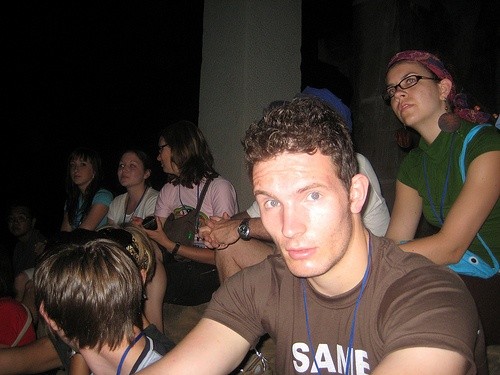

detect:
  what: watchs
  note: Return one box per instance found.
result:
[239,217,251,241]
[64,347,80,358]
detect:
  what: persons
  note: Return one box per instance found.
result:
[141,117,240,334]
[15,145,114,317]
[33,238,177,375]
[36,142,159,340]
[0,223,157,375]
[198,87,391,286]
[1,188,44,300]
[383,49,500,347]
[126,93,488,375]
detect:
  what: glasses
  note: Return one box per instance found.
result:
[382,75,442,102]
[157,144,169,153]
[6,216,32,223]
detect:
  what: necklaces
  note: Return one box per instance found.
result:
[128,192,139,212]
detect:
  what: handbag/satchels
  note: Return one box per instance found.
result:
[400,240,495,279]
[162,204,208,262]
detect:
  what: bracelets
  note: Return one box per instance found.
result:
[171,241,183,255]
